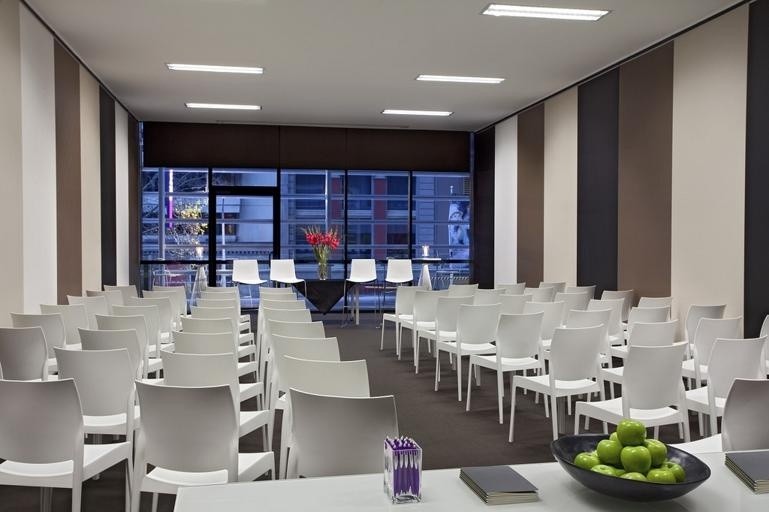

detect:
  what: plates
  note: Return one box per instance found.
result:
[550,433,711,502]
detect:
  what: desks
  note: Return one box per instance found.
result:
[171,449,769,512]
[411,257,442,290]
[292,278,355,328]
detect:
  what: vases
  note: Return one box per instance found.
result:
[318,262,328,281]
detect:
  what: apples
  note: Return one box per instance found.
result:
[574,420,685,484]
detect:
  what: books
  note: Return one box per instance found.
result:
[725,451,769,495]
[459,466,540,506]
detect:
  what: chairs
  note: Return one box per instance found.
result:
[338,258,380,330]
[230,258,267,307]
[381,258,414,314]
[267,258,309,301]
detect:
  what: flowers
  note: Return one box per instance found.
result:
[299,223,343,278]
